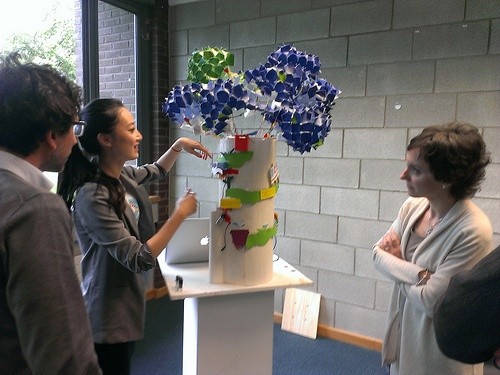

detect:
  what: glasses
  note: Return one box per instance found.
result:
[71,119,87,137]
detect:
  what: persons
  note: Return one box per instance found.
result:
[432,243,499,363]
[0,51,103,375]
[371,121,493,374]
[57,99,213,374]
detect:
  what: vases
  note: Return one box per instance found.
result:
[209,137,278,286]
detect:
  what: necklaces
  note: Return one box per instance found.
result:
[422,211,444,235]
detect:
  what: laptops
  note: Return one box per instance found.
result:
[164,218,209,264]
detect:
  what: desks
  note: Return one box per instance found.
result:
[156,247,313,374]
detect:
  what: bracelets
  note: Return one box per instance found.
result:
[416,270,431,286]
[171,144,183,153]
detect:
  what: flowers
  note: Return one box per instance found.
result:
[163,44,341,156]
